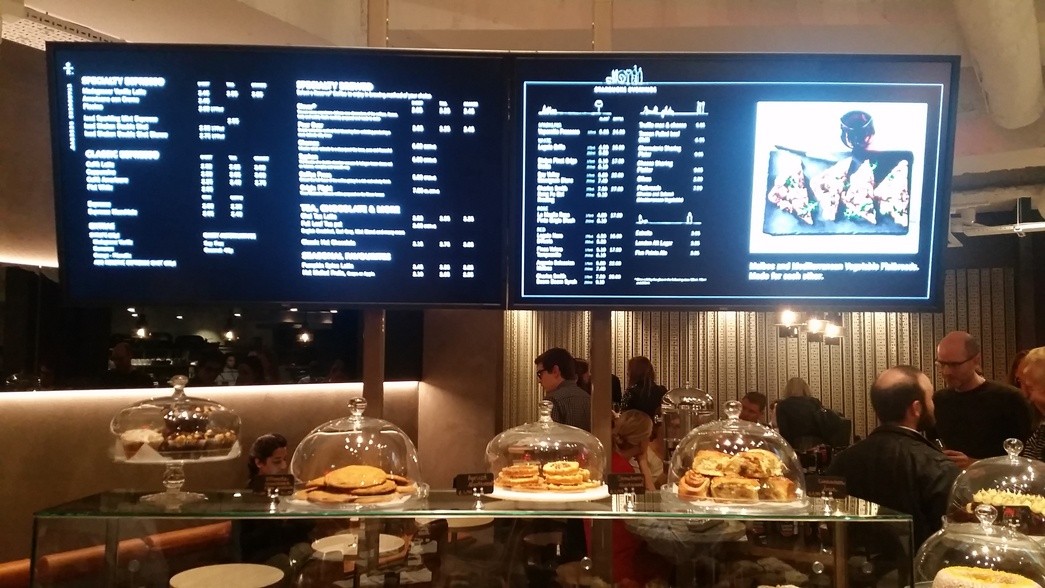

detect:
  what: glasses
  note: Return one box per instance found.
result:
[934,354,976,370]
[536,364,564,381]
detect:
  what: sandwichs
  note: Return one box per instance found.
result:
[767,156,908,226]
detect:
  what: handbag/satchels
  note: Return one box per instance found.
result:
[812,396,843,441]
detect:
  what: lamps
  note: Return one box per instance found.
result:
[773,310,844,347]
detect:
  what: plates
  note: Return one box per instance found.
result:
[413,517,494,528]
[311,534,405,557]
[523,532,564,545]
[763,151,913,235]
[169,563,284,588]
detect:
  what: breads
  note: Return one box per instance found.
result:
[494,461,599,491]
[678,449,792,504]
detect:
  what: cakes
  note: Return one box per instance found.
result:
[966,489,1045,538]
[931,565,1044,588]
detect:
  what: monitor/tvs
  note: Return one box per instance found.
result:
[47,38,509,313]
[510,51,960,315]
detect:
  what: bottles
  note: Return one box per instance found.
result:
[751,521,819,539]
[796,444,849,475]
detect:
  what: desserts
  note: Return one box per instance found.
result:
[119,409,238,460]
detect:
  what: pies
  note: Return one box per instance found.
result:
[296,464,418,503]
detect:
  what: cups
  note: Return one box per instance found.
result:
[841,110,874,152]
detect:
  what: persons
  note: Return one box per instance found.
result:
[228,433,317,565]
[612,355,682,495]
[1006,346,1045,462]
[844,365,958,554]
[740,376,862,498]
[535,348,590,434]
[921,332,1035,472]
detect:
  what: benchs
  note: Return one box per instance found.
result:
[0,522,234,588]
